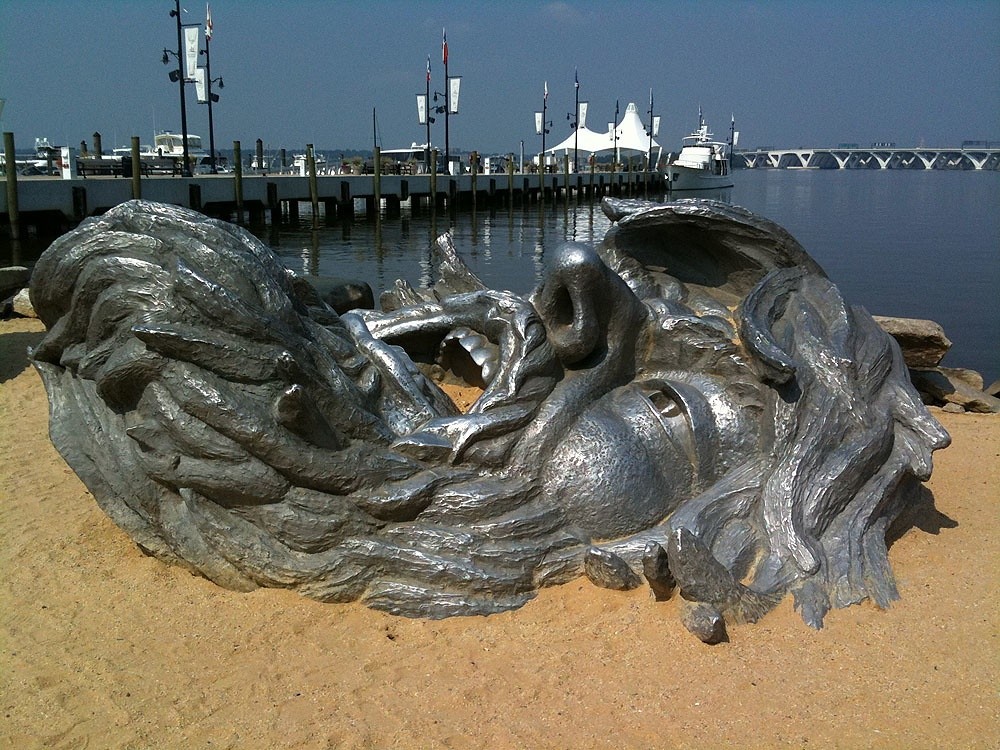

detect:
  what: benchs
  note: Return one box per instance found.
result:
[76,158,124,178]
[140,158,183,178]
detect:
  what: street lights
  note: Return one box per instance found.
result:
[611,100,622,163]
[567,67,580,174]
[540,80,553,155]
[726,114,734,168]
[434,29,458,175]
[196,2,225,175]
[642,88,657,171]
[423,54,435,175]
[160,0,202,178]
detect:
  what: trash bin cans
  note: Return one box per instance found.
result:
[121,156,133,177]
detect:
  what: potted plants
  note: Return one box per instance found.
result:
[340,157,364,175]
[406,160,417,175]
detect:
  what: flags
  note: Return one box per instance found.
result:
[426,54,431,82]
[205,3,213,41]
[575,67,579,88]
[615,97,619,114]
[443,27,449,65]
[650,88,654,104]
[731,113,735,126]
[544,81,548,101]
[698,104,702,118]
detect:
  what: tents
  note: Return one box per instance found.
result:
[539,103,660,174]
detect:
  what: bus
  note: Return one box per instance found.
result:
[871,141,895,148]
[732,148,749,153]
[838,142,858,149]
[757,144,776,151]
[961,141,987,148]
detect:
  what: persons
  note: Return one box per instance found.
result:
[32,196,952,646]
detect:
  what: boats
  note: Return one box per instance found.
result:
[667,120,736,190]
